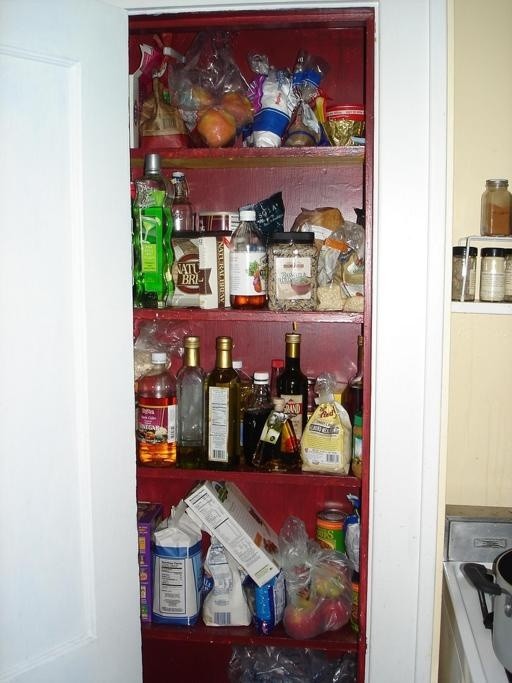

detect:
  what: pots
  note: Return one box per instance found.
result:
[462,548,512,673]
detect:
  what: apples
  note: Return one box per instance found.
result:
[219,93,251,124]
[315,565,347,601]
[286,564,311,601]
[284,600,322,639]
[322,595,352,630]
[190,85,212,115]
[198,106,237,146]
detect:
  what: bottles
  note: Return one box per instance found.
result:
[480,247,507,305]
[179,336,209,466]
[319,508,349,557]
[480,177,511,239]
[134,152,173,309]
[137,355,178,466]
[173,172,193,233]
[208,335,240,471]
[454,244,478,302]
[234,361,254,403]
[227,210,266,308]
[269,227,320,310]
[250,374,270,447]
[268,358,284,394]
[342,332,366,475]
[279,330,308,451]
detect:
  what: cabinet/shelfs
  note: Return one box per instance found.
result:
[451,235,512,314]
[438,590,465,683]
[127,6,374,682]
